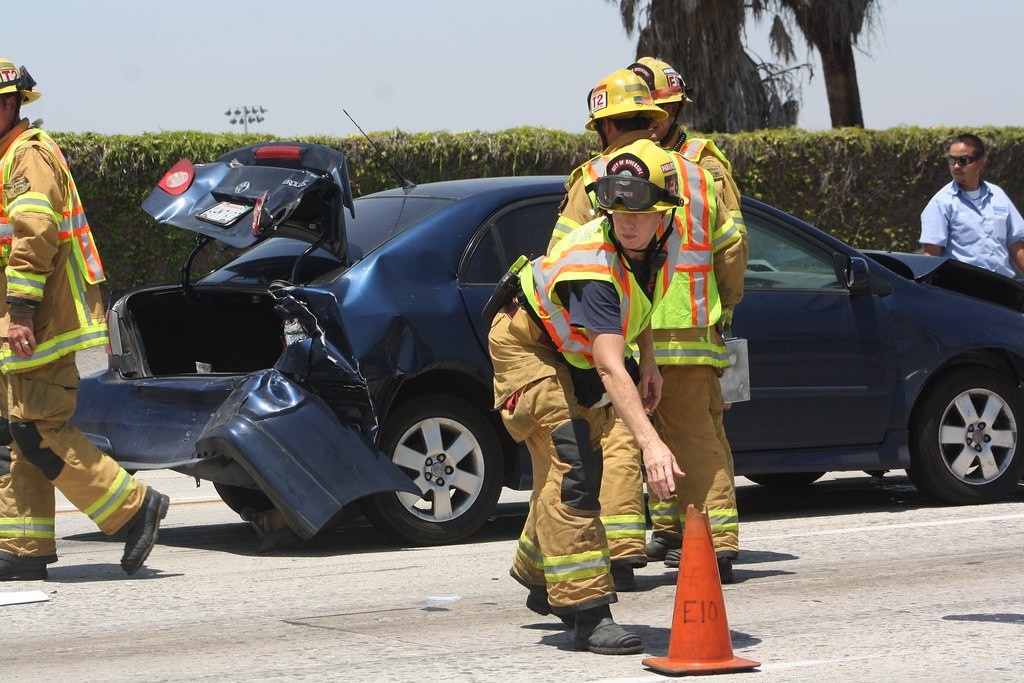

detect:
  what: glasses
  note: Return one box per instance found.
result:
[946,154,980,166]
[594,176,669,211]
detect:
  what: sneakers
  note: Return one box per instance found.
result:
[717,558,734,583]
[0,559,48,580]
[575,605,644,655]
[664,548,682,567]
[645,540,678,562]
[526,590,575,629]
[610,564,636,591]
[121,488,170,575]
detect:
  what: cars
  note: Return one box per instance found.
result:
[71,141,1024,549]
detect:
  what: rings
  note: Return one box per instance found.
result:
[22,341,28,347]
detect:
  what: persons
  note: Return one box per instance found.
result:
[919,134,1024,282]
[547,56,748,582]
[0,57,170,582]
[484,175,686,653]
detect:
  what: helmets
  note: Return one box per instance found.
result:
[585,70,669,131]
[596,139,690,213]
[626,57,693,105]
[0,58,41,106]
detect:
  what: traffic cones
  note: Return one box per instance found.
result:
[638,501,762,676]
